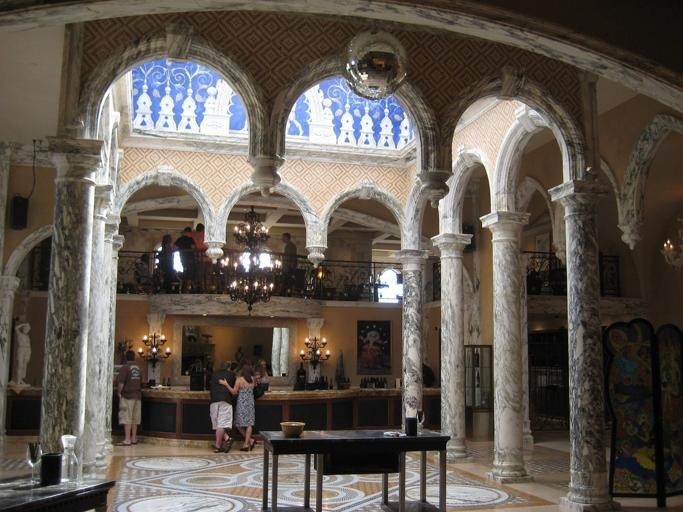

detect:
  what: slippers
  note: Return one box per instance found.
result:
[114,439,141,447]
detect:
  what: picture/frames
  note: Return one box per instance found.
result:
[355,319,393,377]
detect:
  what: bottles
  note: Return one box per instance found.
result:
[314,376,386,390]
[161,377,171,390]
[59,434,78,483]
[296,361,306,391]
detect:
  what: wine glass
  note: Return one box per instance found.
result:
[25,439,41,485]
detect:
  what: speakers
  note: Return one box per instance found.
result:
[463,225,476,253]
[10,196,28,231]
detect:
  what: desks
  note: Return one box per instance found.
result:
[259,429,451,512]
[0,473,116,512]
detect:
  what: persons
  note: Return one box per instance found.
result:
[134,253,152,285]
[218,363,259,452]
[422,361,435,386]
[115,349,143,447]
[13,322,32,386]
[206,362,235,454]
[361,330,388,372]
[235,258,244,286]
[280,232,298,296]
[256,358,268,376]
[172,226,196,294]
[159,234,174,293]
[177,222,209,293]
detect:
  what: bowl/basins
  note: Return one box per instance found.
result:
[279,421,306,438]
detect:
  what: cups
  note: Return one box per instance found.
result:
[405,416,417,436]
[41,451,61,486]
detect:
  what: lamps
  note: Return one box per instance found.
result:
[138,332,172,372]
[660,217,683,272]
[300,336,331,374]
[220,205,281,307]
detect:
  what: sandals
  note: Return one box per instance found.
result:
[213,437,233,452]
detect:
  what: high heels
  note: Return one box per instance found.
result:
[240,440,257,451]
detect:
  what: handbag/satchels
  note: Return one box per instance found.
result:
[254,384,264,398]
